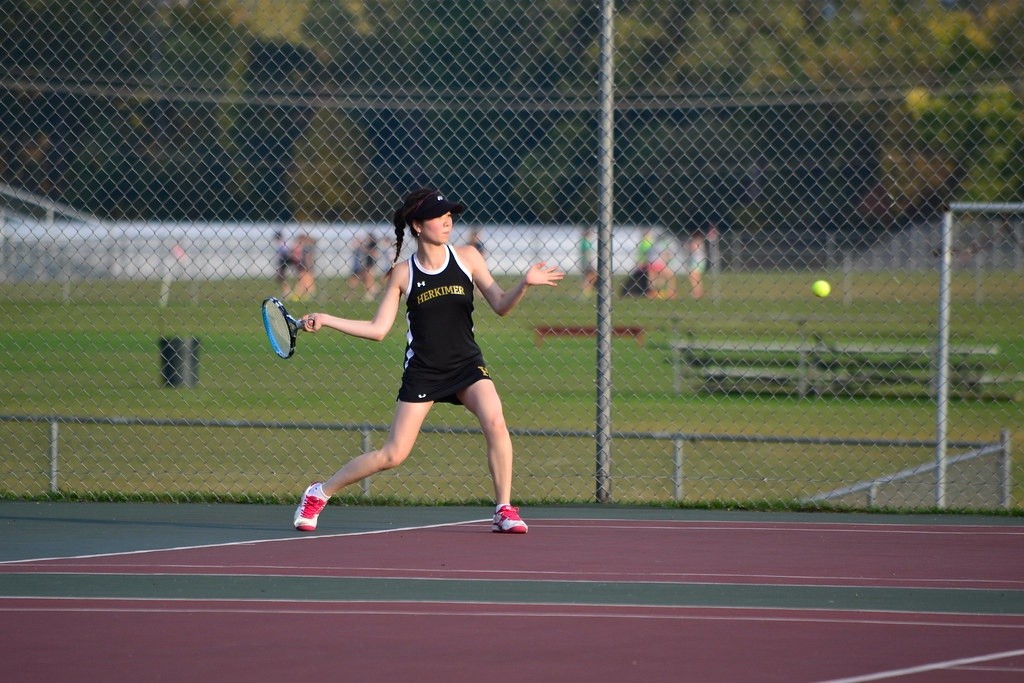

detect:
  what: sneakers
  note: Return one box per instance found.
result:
[491,505,529,534]
[294,481,327,531]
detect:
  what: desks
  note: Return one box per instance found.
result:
[674,339,1002,394]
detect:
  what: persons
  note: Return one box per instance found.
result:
[277,232,316,302]
[580,230,597,297]
[637,227,718,298]
[343,234,396,301]
[469,233,484,254]
[295,191,564,533]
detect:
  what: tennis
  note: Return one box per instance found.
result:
[812,279,831,299]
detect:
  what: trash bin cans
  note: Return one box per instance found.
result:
[160,336,200,386]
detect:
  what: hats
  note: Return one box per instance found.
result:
[407,191,464,226]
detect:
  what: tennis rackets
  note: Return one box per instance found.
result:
[260,297,315,359]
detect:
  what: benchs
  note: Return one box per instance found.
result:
[686,357,1024,400]
